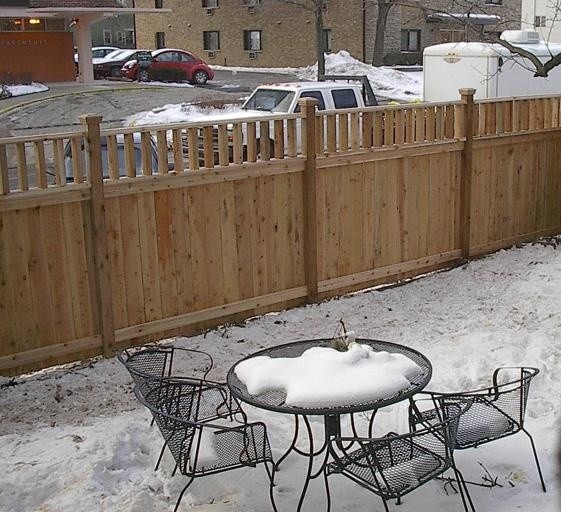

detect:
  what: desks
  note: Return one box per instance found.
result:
[225,338,432,512]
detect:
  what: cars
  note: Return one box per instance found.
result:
[42,126,180,191]
[73,44,216,85]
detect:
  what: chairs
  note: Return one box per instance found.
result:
[133,375,280,512]
[407,367,546,512]
[323,390,477,512]
[118,345,248,471]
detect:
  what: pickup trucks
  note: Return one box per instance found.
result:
[166,71,455,167]
